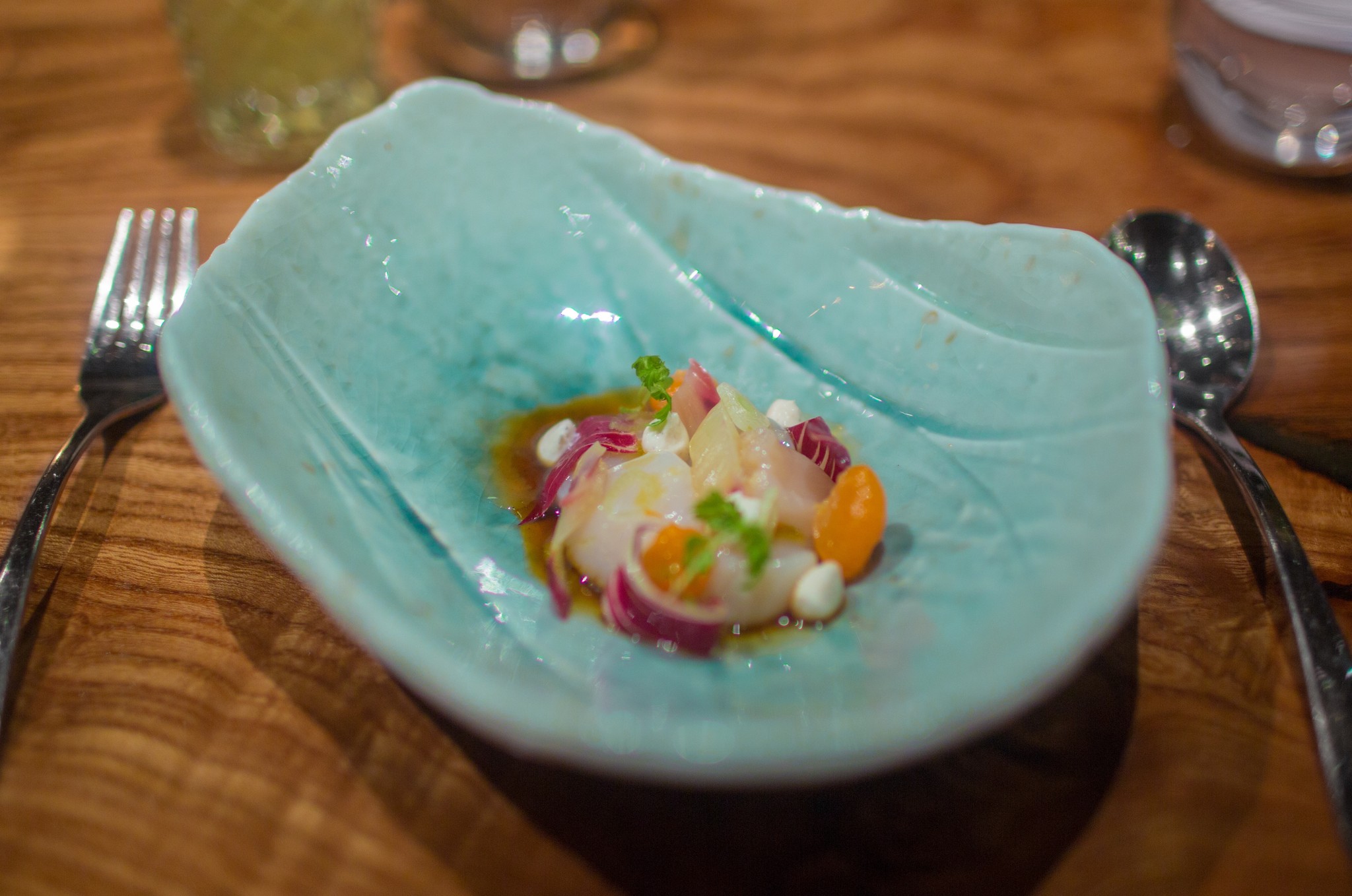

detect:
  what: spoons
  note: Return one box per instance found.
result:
[1098,203,1350,842]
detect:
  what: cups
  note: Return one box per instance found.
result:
[1164,3,1349,186]
[415,0,662,91]
[155,2,388,171]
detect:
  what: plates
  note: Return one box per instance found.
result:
[145,76,1171,791]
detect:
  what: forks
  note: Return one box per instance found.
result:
[1,204,202,712]
